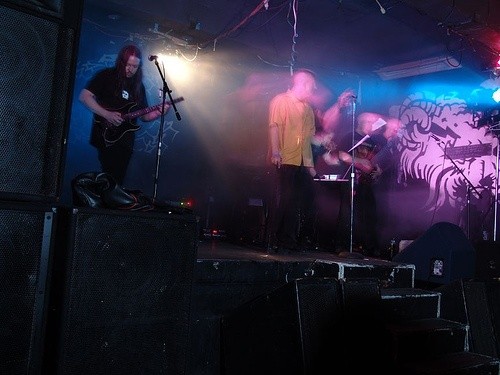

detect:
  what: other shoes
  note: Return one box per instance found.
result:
[267,236,280,249]
[304,241,320,251]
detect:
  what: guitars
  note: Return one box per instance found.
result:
[94,96,184,144]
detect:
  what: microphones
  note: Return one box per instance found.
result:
[148,56,158,61]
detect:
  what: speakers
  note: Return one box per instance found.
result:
[1,0,76,199]
[220,279,341,375]
[339,277,379,374]
[51,206,195,374]
[0,210,57,375]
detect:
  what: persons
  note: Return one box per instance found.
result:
[269,69,351,254]
[217,71,266,160]
[78,44,171,187]
[339,113,404,254]
